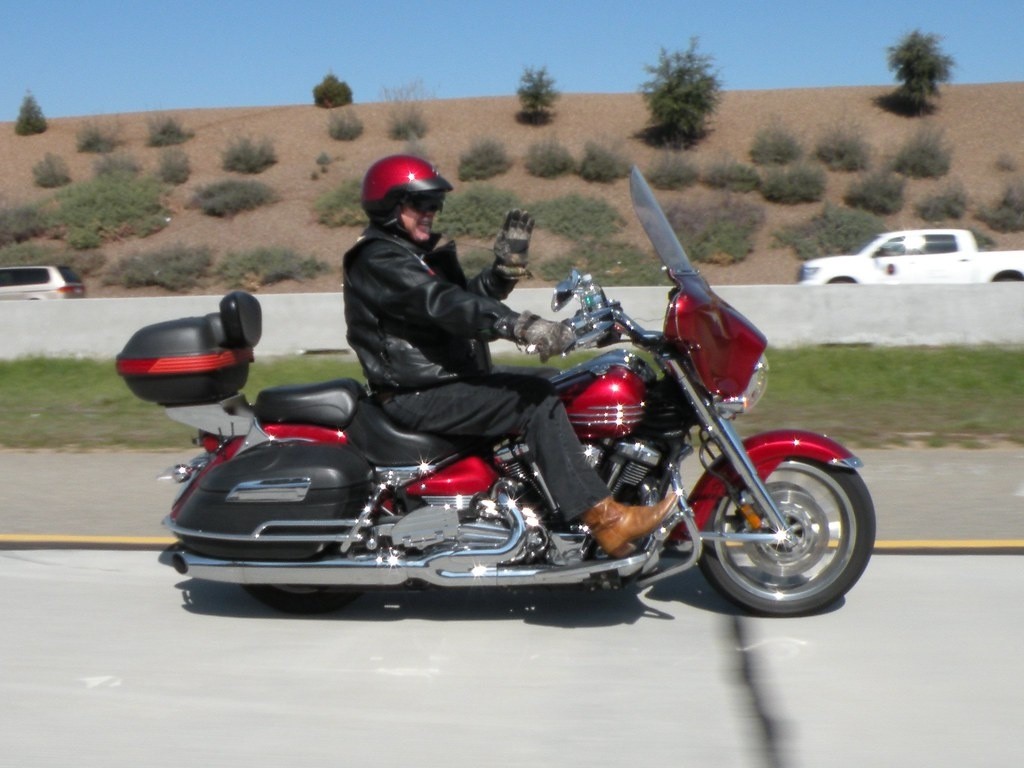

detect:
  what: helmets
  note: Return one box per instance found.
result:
[361,155,455,214]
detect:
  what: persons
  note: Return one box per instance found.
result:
[340,155,679,559]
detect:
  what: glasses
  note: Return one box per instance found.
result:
[405,193,440,214]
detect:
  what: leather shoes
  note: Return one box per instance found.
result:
[580,492,679,559]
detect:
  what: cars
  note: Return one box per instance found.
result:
[0,265,86,301]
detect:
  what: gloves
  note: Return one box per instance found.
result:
[514,310,577,363]
[494,207,534,280]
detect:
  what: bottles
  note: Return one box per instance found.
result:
[582,274,614,322]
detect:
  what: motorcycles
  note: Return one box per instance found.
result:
[113,164,876,620]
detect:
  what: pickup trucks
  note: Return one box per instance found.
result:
[798,228,1024,285]
